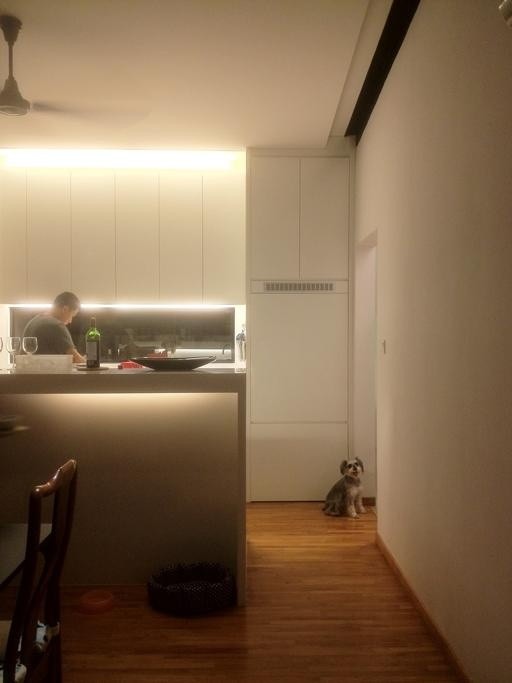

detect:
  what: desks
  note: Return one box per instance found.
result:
[0,521,52,586]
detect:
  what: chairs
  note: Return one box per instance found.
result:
[0,459,78,682]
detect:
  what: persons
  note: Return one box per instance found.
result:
[16,290,87,363]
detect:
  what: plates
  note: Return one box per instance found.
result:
[130,356,216,370]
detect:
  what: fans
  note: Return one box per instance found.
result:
[0,15,92,118]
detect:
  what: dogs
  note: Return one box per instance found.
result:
[322,455,367,519]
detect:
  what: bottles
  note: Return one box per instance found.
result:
[235,328,246,373]
[86,318,100,368]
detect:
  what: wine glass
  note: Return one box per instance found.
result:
[0,337,37,370]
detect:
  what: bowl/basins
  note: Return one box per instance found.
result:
[75,588,116,615]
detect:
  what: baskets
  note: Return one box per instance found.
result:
[146,560,233,617]
